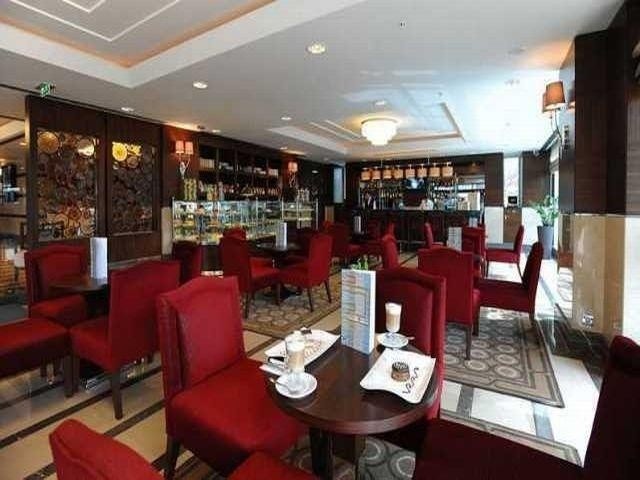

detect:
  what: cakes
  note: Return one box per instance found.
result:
[391,362,410,382]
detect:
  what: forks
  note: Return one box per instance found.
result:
[268,376,298,394]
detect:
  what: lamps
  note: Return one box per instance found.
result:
[361,155,453,181]
[288,162,298,188]
[361,117,398,147]
[542,81,565,145]
[176,140,194,179]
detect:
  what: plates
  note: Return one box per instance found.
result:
[376,332,412,347]
[359,348,438,404]
[273,373,320,399]
[261,326,342,371]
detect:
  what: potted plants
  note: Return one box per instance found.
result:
[528,194,560,260]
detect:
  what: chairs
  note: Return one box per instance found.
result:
[411,334,640,479]
[170,240,202,285]
[424,223,485,249]
[417,246,482,361]
[364,265,449,453]
[223,227,281,318]
[276,233,335,313]
[485,225,525,277]
[155,276,315,478]
[70,259,181,424]
[1,317,70,399]
[217,223,393,268]
[50,418,309,478]
[22,244,91,378]
[475,241,545,336]
[381,234,417,270]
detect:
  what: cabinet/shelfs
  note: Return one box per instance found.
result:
[196,132,283,200]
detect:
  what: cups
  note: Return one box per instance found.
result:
[385,301,403,334]
[284,334,308,373]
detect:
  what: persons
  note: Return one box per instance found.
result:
[418,195,434,210]
[360,190,377,210]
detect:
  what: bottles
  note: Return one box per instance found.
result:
[251,186,279,196]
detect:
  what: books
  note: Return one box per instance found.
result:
[468,217,478,228]
[87,234,112,282]
[338,268,378,355]
[276,221,289,246]
[353,216,362,234]
[447,226,463,255]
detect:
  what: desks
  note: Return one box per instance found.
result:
[265,334,440,480]
[49,271,111,379]
